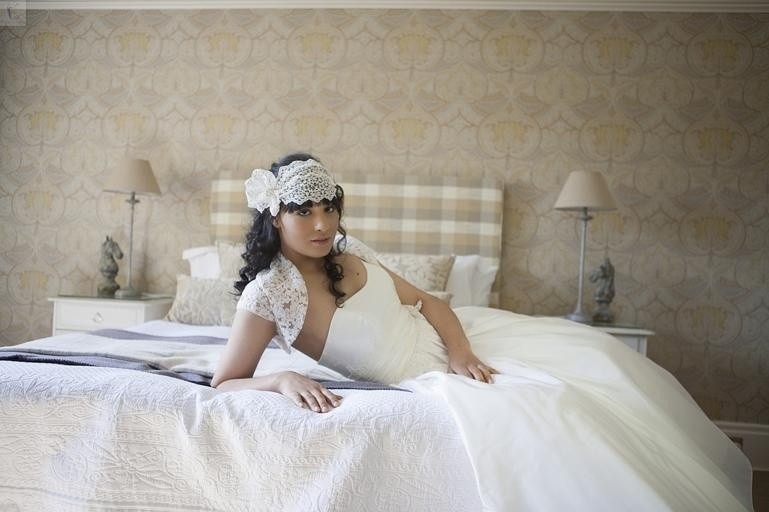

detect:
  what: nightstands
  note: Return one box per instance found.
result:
[46,292,175,338]
[545,318,658,359]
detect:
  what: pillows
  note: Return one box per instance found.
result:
[214,238,246,279]
[477,258,502,306]
[181,248,217,277]
[421,290,454,307]
[444,255,478,307]
[379,254,456,291]
[166,273,248,329]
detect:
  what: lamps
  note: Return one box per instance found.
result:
[101,157,163,298]
[554,170,617,329]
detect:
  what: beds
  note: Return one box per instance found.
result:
[2,176,753,511]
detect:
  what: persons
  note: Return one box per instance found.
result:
[206,153,756,511]
